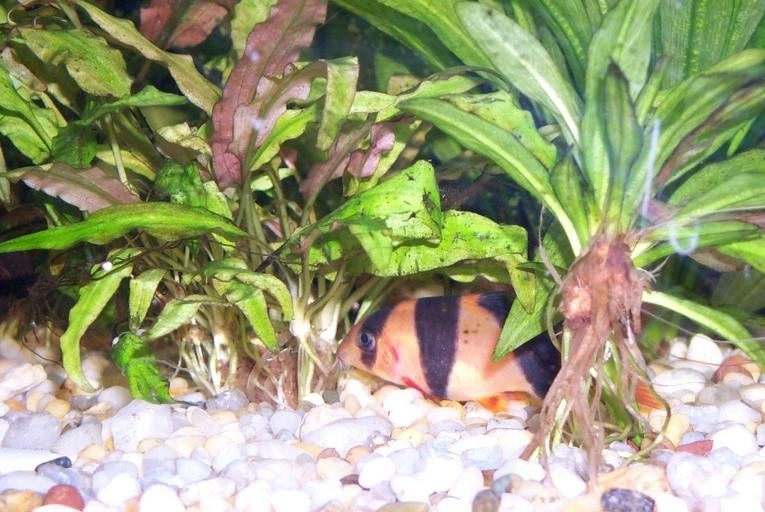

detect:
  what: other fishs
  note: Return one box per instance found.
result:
[334,289,666,417]
